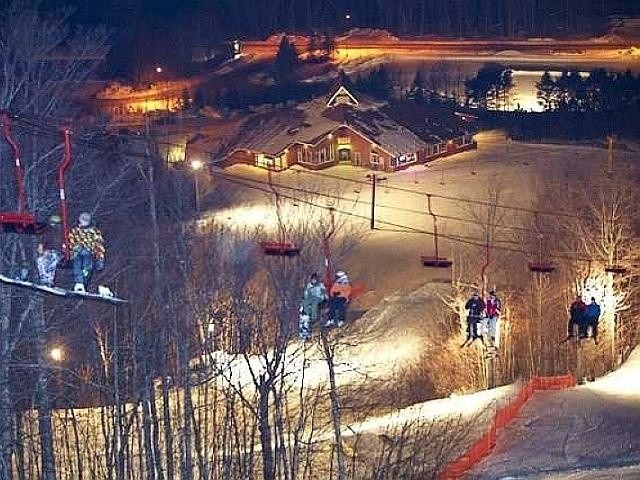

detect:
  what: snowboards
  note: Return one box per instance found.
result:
[69,290,126,304]
[1,274,65,296]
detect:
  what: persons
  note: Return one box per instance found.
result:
[62,210,106,291]
[34,215,65,288]
[324,269,353,329]
[463,293,486,338]
[297,271,329,337]
[567,295,586,336]
[482,290,502,344]
[582,297,601,338]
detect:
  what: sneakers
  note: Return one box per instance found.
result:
[97,285,110,297]
[73,283,86,292]
